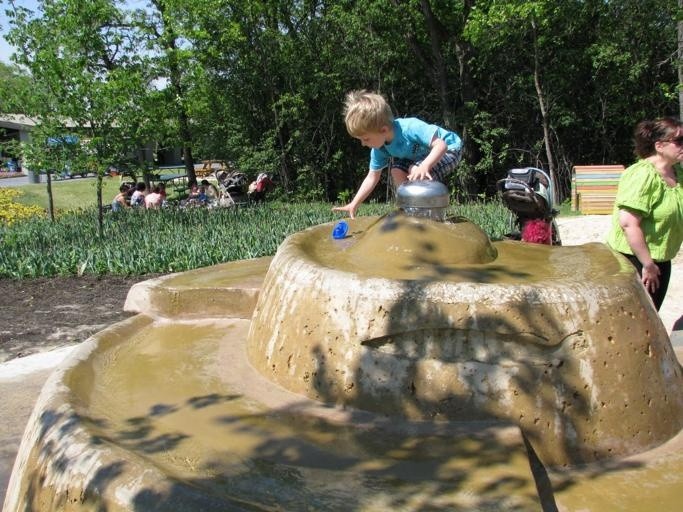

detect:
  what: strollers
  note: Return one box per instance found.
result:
[214,170,273,212]
[496,167,560,246]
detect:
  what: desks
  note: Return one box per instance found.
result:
[150,159,226,188]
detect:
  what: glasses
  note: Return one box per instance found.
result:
[657,136,682,145]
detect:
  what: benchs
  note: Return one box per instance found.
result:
[570,165,626,215]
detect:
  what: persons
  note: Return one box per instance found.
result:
[606,116,683,314]
[112,182,166,213]
[328,88,465,221]
[181,180,218,207]
